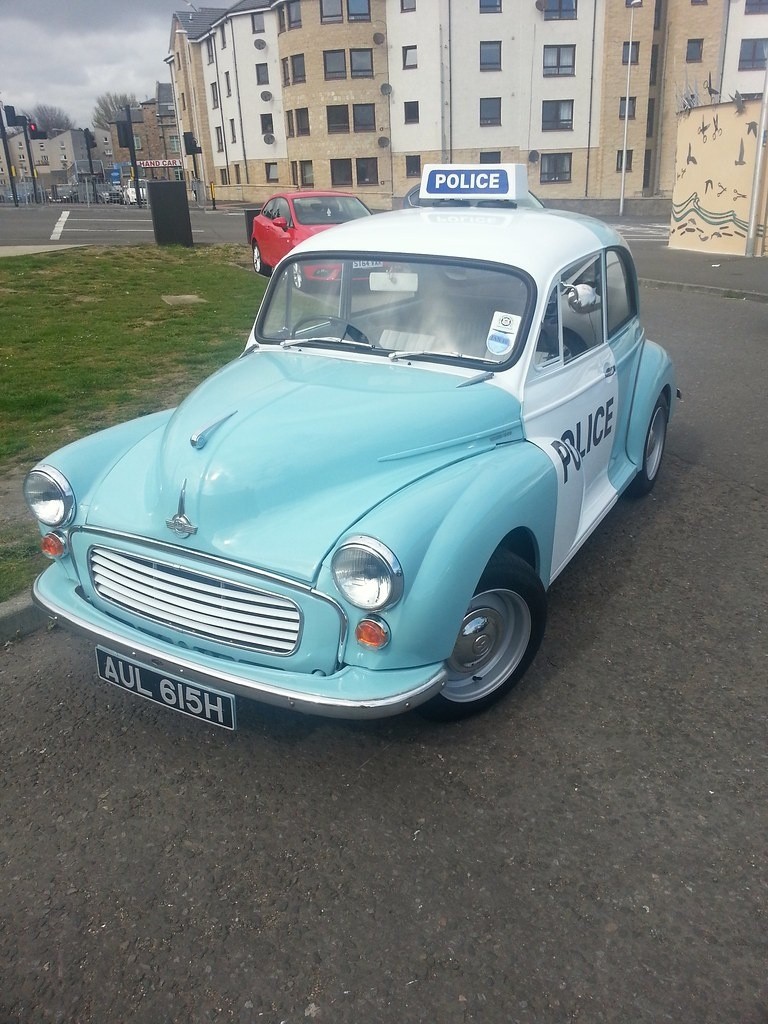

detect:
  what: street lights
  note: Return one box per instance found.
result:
[616,0,643,217]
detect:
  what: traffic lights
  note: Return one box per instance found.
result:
[27,122,38,138]
[184,132,203,155]
[84,128,98,150]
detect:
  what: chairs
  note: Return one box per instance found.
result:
[415,276,559,355]
[294,202,342,219]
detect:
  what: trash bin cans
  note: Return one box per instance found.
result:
[243,208,262,244]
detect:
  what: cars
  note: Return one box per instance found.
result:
[252,190,402,292]
[22,162,676,732]
[122,179,150,205]
[96,183,123,203]
